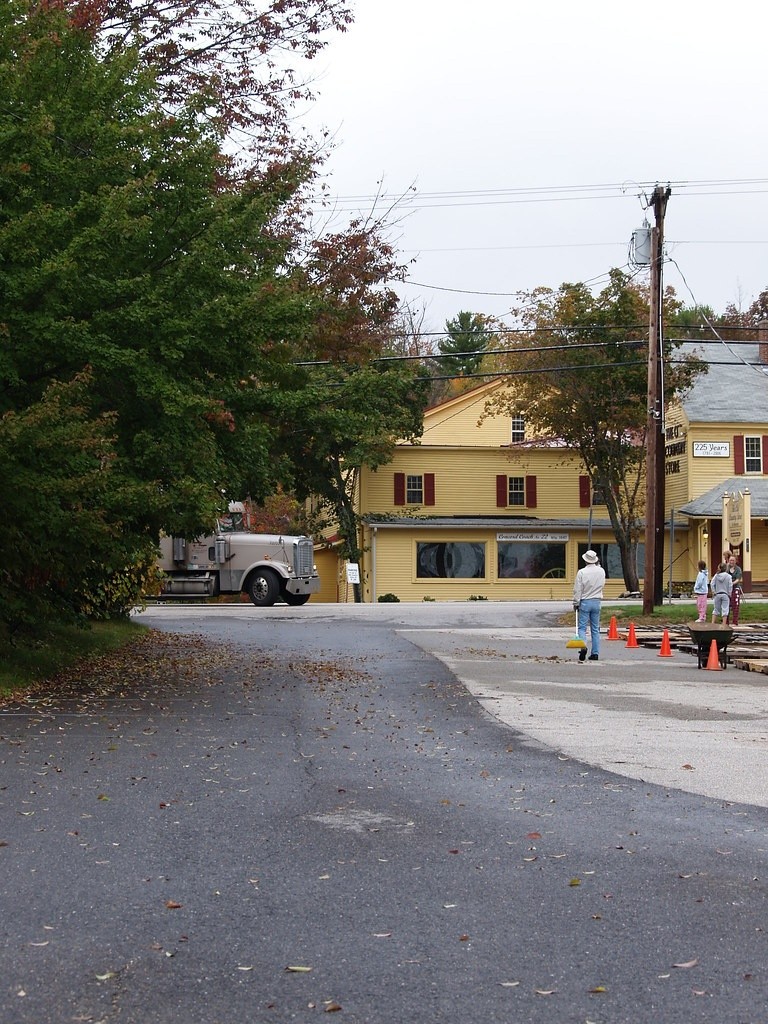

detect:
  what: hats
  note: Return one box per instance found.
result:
[582,550,599,564]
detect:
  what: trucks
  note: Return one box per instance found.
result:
[136,498,320,607]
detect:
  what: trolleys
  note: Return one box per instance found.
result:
[686,622,739,670]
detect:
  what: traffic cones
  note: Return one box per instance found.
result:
[604,616,621,640]
[702,639,723,671]
[624,621,640,648]
[657,629,676,658]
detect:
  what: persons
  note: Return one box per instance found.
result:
[727,554,742,626]
[693,560,708,623]
[710,563,733,625]
[573,550,605,661]
[723,551,732,573]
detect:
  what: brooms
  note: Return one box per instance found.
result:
[566,609,587,648]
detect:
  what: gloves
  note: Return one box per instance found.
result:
[574,605,580,612]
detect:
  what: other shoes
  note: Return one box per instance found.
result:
[588,654,598,660]
[695,619,704,623]
[578,648,587,661]
